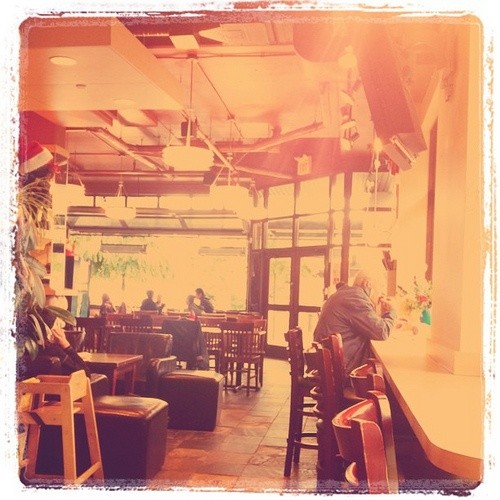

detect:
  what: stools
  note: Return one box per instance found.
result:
[158,370,224,431]
[285,326,399,494]
[93,394,169,481]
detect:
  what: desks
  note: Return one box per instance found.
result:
[201,327,266,392]
[76,353,144,396]
[370,325,484,482]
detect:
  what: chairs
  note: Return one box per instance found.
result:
[65,310,266,396]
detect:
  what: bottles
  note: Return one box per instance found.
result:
[190,311,195,320]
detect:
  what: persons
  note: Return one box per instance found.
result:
[193,288,214,312]
[186,295,202,316]
[24,307,91,378]
[313,268,396,384]
[141,290,167,315]
[100,293,115,315]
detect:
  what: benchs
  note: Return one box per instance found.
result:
[106,331,176,396]
